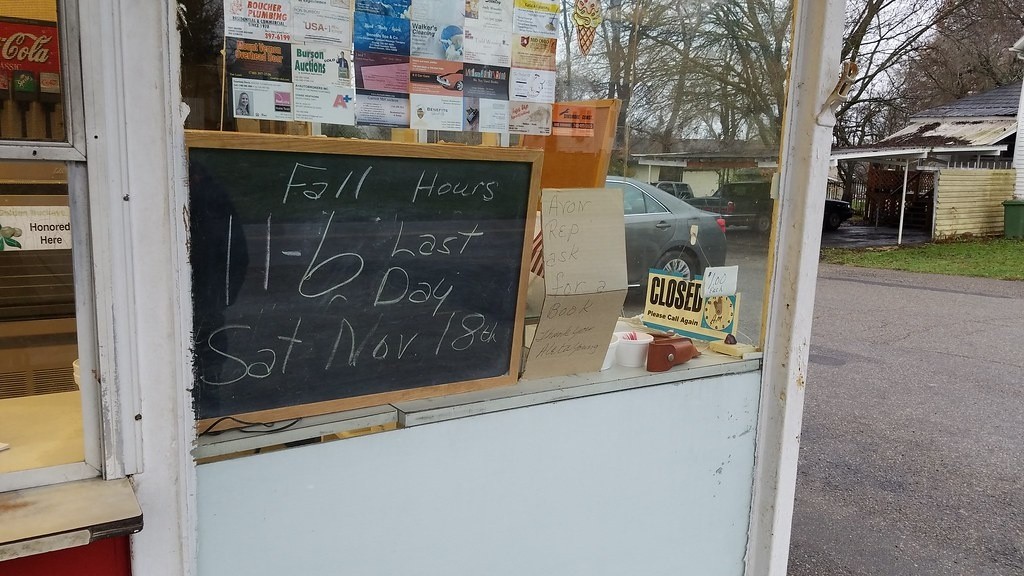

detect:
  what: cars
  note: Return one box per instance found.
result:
[685,181,852,231]
[602,172,728,297]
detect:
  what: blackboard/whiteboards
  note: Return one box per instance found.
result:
[182,126,546,437]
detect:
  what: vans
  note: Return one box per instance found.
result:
[645,181,694,200]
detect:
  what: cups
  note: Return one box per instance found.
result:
[0,99,66,142]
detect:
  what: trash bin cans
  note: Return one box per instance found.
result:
[1002,200,1024,240]
